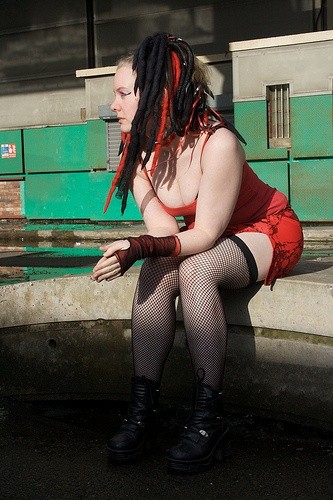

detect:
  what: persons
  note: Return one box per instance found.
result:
[89,32,304,472]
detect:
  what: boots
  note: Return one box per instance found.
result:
[103,373,169,462]
[160,367,238,473]
